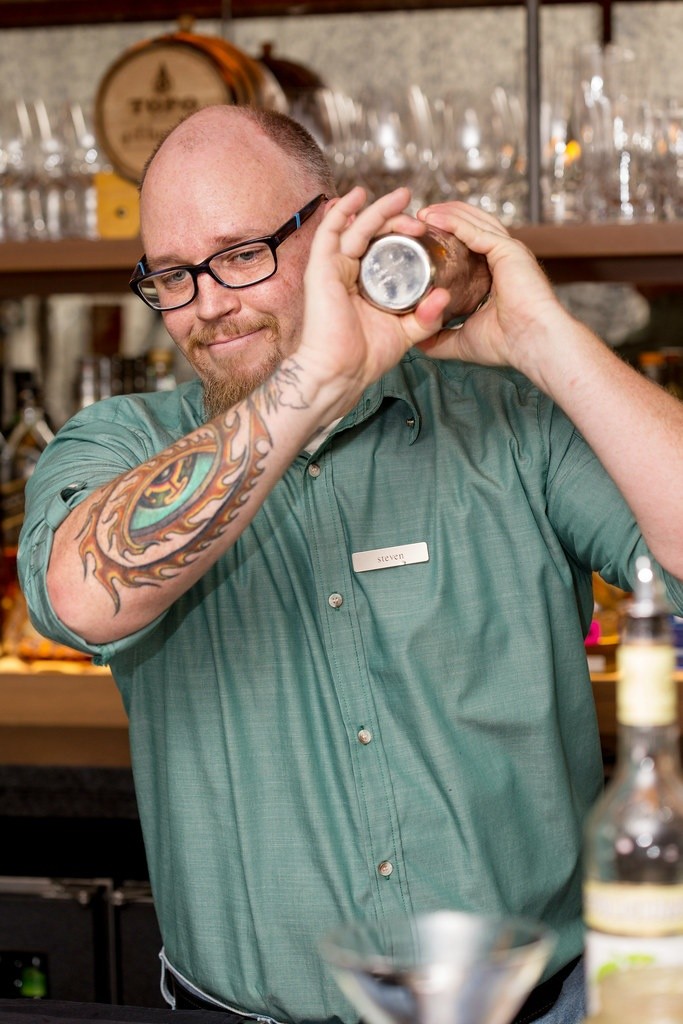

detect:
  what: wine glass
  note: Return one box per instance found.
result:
[291,83,528,228]
[538,45,683,225]
[0,102,107,240]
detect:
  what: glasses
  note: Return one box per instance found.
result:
[130,194,325,311]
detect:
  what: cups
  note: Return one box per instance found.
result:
[361,224,492,324]
[318,909,558,1024]
[600,966,683,1024]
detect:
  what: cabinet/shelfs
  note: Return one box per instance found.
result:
[0,152,683,770]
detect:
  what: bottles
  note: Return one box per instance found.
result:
[0,295,177,583]
[582,559,683,1024]
[640,346,683,401]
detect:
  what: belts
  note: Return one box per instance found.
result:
[162,958,583,1024]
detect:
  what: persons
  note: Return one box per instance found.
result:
[15,102,683,1024]
